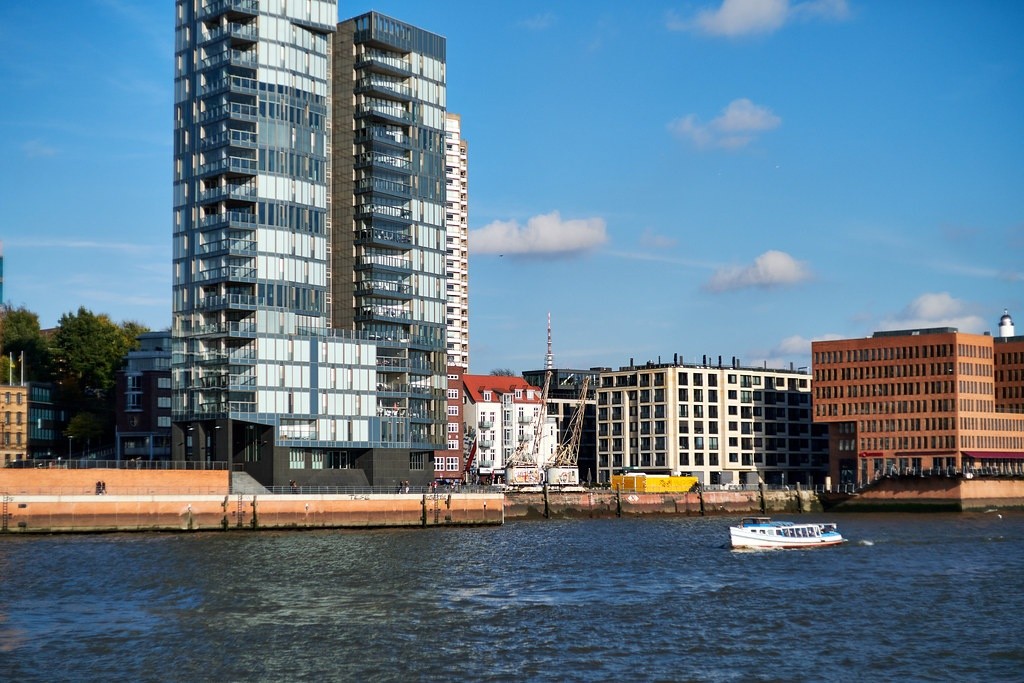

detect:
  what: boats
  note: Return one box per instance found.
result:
[729,517,848,549]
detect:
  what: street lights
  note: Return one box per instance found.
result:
[67,431,74,469]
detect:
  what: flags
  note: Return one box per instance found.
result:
[18,355,22,362]
[11,359,15,368]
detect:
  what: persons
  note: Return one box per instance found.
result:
[398,480,410,494]
[427,481,437,493]
[289,480,298,494]
[451,480,455,489]
[460,479,466,492]
[96,481,107,495]
